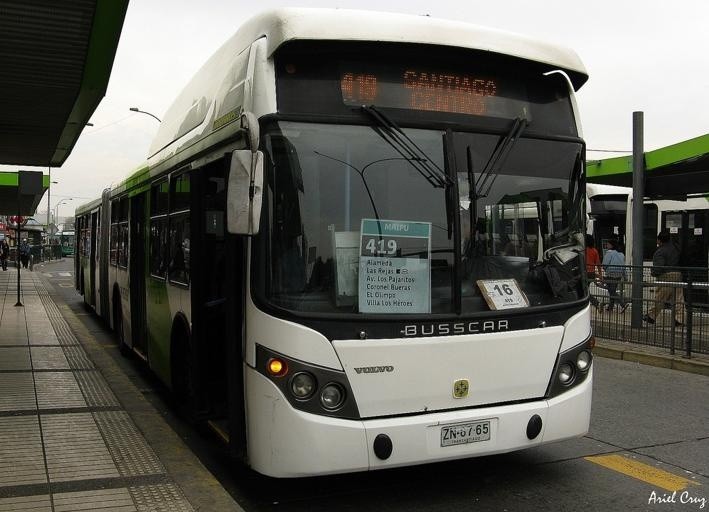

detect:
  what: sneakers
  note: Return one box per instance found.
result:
[675,319,683,327]
[619,303,629,314]
[605,307,613,311]
[598,303,604,314]
[643,313,655,324]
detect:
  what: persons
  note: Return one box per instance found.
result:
[0,240,10,272]
[20,239,30,268]
[601,239,630,313]
[585,233,604,314]
[643,229,686,328]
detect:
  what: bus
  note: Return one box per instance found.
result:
[55,231,75,257]
[460,183,709,291]
[74,6,595,478]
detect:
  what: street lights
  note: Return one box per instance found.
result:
[51,181,58,183]
[129,107,161,124]
[54,199,72,227]
